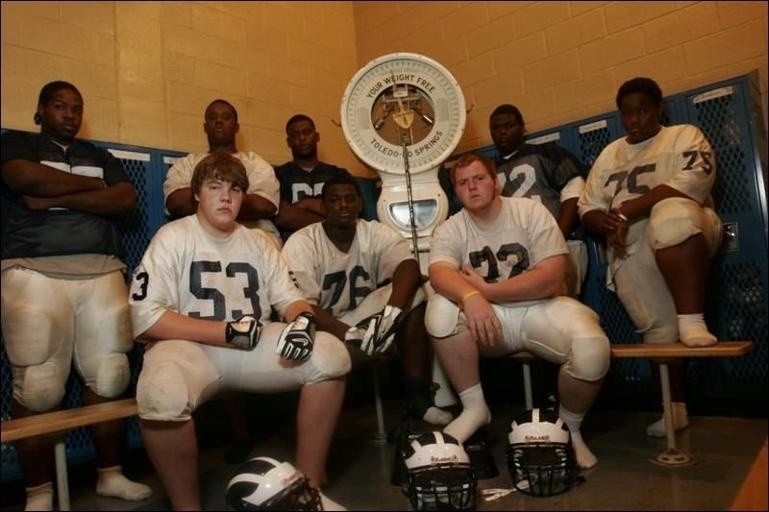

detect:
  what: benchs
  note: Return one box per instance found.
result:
[2,353,390,511]
[418,338,754,474]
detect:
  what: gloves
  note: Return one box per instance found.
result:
[225,313,263,350]
[361,305,405,357]
[274,311,318,362]
[344,324,404,362]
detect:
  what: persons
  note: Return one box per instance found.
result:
[425,151,613,472]
[274,110,367,245]
[577,75,725,440]
[1,78,154,512]
[424,104,587,428]
[161,100,283,463]
[128,150,355,512]
[278,173,418,487]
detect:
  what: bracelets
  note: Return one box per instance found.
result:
[619,211,630,223]
[459,288,480,312]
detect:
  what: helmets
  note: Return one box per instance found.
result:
[223,455,311,511]
[397,430,475,510]
[507,407,573,484]
